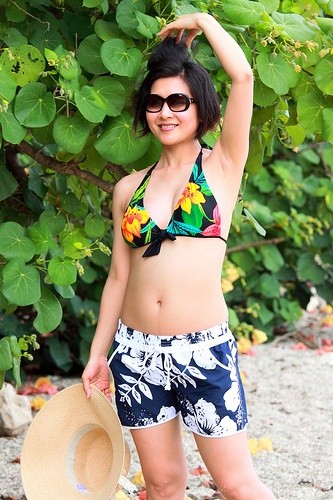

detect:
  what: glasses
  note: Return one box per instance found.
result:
[143,93,196,114]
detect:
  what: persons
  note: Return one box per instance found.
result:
[80,11,276,499]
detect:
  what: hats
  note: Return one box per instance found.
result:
[19,381,131,499]
